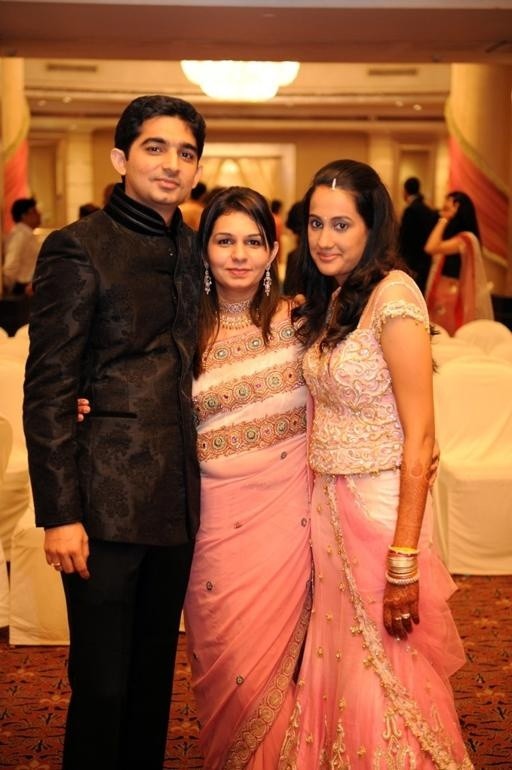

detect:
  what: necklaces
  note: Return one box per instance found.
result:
[216,296,255,330]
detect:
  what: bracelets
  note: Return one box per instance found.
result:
[385,543,420,586]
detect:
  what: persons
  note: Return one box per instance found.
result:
[178,183,304,296]
[23,96,208,770]
[79,204,101,219]
[0,196,43,337]
[398,176,494,336]
[279,159,476,769]
[77,185,315,770]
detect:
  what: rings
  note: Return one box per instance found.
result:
[394,617,402,621]
[401,613,411,620]
[54,563,61,568]
